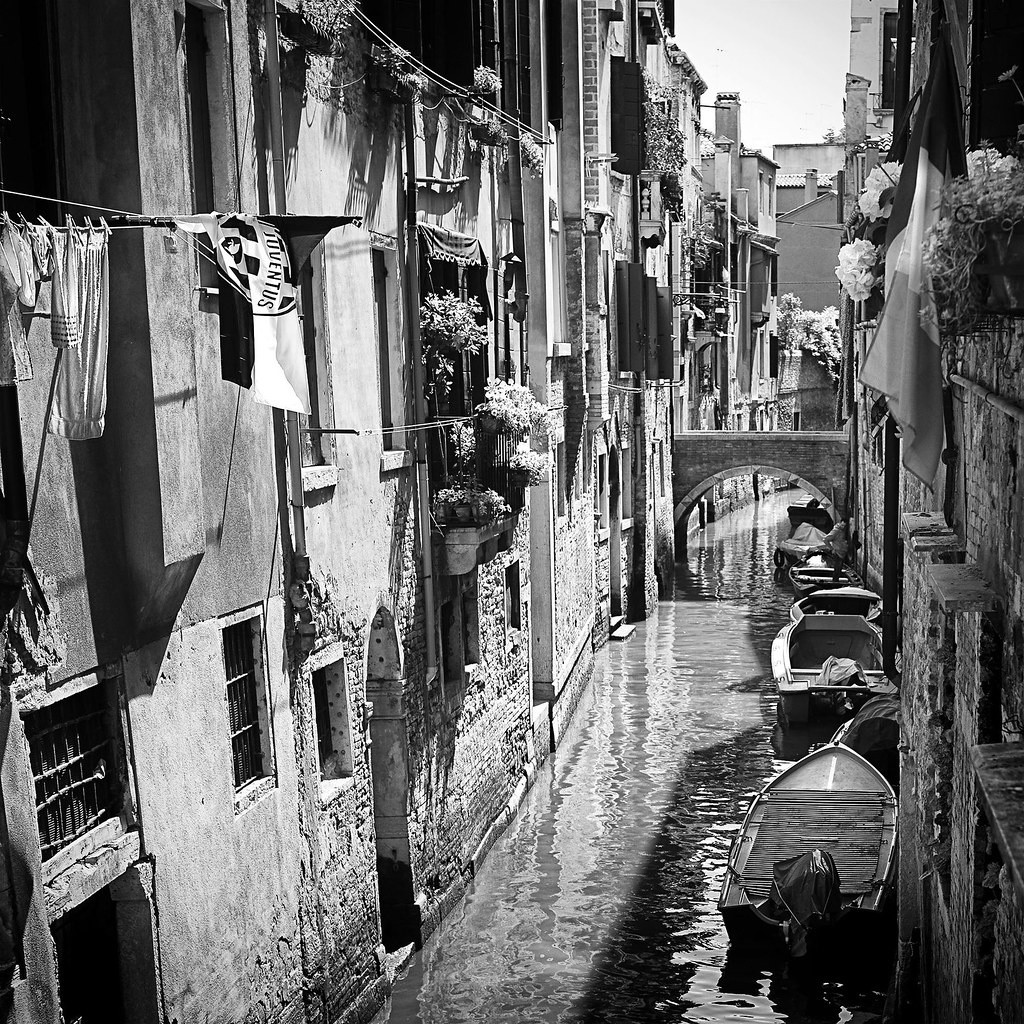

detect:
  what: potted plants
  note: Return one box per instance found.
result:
[471,119,501,144]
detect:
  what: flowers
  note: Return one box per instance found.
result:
[966,148,1019,180]
[509,441,555,487]
[858,159,904,223]
[834,236,877,302]
[475,378,548,432]
[436,485,513,523]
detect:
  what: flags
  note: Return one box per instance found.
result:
[854,9,970,499]
[169,214,367,415]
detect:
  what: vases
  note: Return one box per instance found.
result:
[456,505,470,522]
[481,416,497,431]
[515,474,530,487]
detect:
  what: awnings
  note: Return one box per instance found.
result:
[415,223,487,275]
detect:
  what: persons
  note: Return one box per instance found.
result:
[823,519,848,582]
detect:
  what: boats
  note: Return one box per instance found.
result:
[767,521,903,725]
[828,692,901,788]
[786,491,829,527]
[714,739,899,965]
